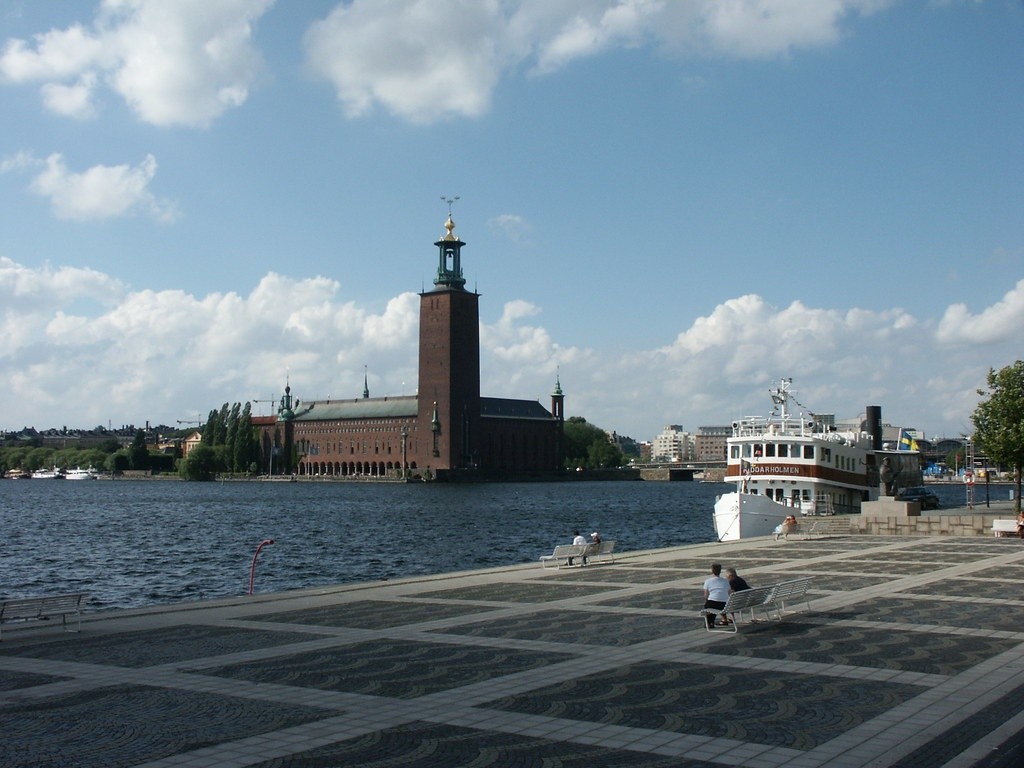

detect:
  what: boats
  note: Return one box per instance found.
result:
[31,465,61,479]
[3,469,29,479]
[712,375,877,542]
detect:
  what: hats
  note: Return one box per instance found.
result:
[591,533,600,539]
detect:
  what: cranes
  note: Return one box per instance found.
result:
[176,414,208,427]
[65,464,99,481]
[252,392,283,417]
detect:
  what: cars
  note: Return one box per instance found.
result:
[895,485,940,511]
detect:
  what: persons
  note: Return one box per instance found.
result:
[1015,511,1024,539]
[564,531,601,566]
[703,564,753,628]
[773,515,796,540]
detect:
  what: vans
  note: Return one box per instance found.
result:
[978,470,998,480]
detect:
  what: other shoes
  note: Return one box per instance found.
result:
[715,621,728,626]
[705,623,714,628]
[727,619,733,623]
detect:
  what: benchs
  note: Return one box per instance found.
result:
[698,576,817,634]
[0,592,90,633]
[991,519,1018,537]
[540,541,618,570]
[805,520,832,538]
[772,523,811,541]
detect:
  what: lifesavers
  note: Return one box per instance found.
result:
[962,471,976,487]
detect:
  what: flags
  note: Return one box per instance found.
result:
[896,430,920,451]
[740,459,751,469]
